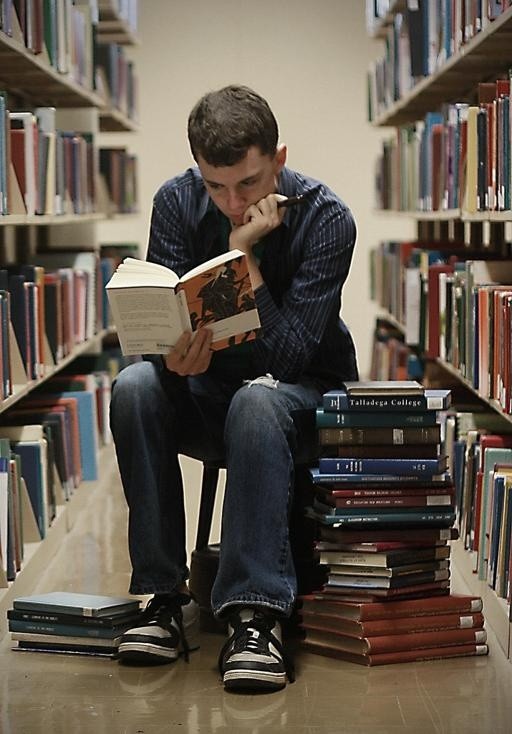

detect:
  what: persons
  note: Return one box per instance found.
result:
[109,85,357,694]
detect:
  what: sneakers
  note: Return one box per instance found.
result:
[118,591,200,665]
[221,610,287,689]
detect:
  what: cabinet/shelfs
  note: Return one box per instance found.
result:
[362,3,512,668]
[2,0,149,642]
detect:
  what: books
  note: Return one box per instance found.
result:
[105,247,262,357]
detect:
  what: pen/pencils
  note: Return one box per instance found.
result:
[278,194,305,208]
